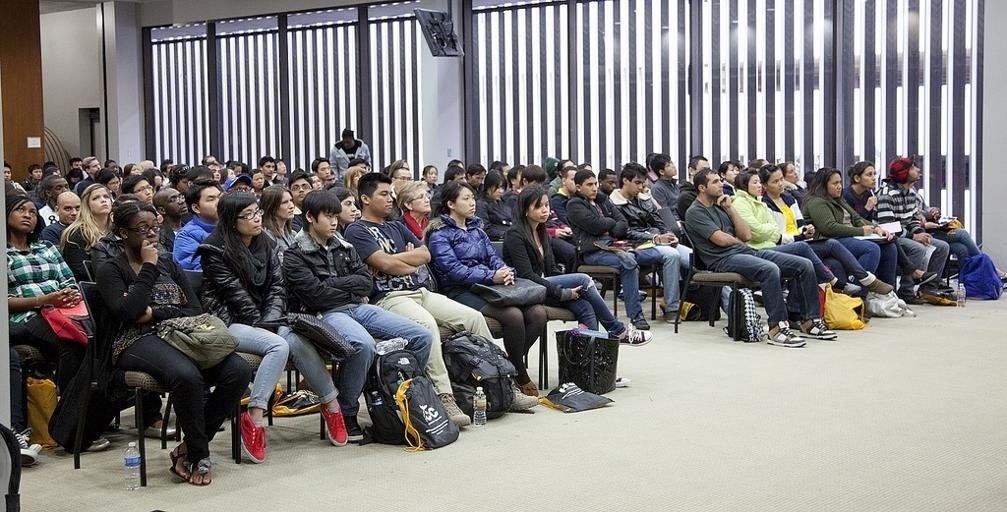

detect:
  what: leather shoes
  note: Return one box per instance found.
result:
[833,270,954,304]
[144,426,178,439]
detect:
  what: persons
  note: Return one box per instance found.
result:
[642,153,1007,348]
[327,129,372,188]
[3,153,661,486]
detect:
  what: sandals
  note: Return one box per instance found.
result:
[170,446,211,485]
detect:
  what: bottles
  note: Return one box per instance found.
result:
[124,442,141,489]
[956,282,965,308]
[472,386,486,425]
[376,337,408,355]
[371,391,382,405]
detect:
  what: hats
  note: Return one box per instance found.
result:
[224,175,252,191]
[890,157,915,183]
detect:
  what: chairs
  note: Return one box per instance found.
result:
[0,421,27,512]
[4,164,959,488]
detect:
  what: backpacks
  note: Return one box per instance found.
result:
[959,254,1004,300]
[441,329,518,419]
[728,288,764,342]
[359,349,460,451]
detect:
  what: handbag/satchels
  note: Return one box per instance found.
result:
[824,284,906,330]
[556,330,619,395]
[40,299,96,344]
[471,278,547,307]
[156,312,239,369]
[254,312,356,358]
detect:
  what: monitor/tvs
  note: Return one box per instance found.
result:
[413,7,464,58]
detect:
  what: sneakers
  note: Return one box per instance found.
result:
[664,311,680,324]
[766,318,837,347]
[624,287,647,300]
[11,427,41,466]
[511,381,539,410]
[438,394,472,426]
[234,412,266,464]
[609,313,652,345]
[321,404,364,446]
[88,439,109,451]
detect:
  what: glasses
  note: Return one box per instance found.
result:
[130,225,161,233]
[238,210,264,220]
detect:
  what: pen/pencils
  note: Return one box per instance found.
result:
[860,219,866,226]
[870,188,875,197]
